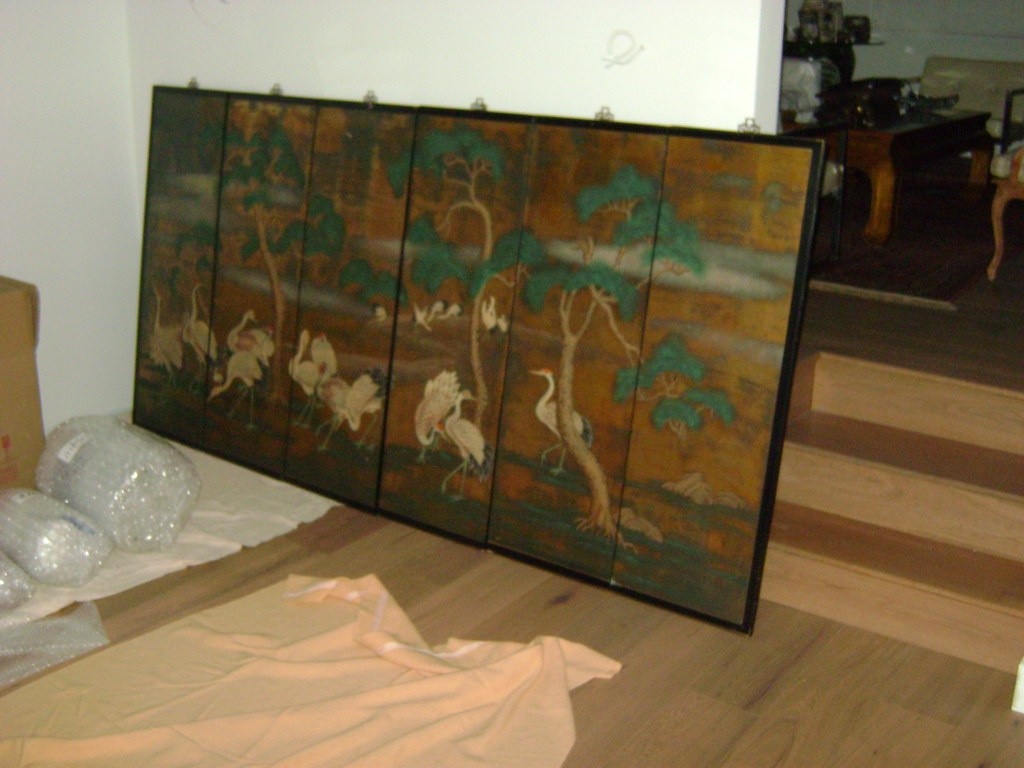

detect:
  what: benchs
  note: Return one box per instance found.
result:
[899,55,1024,158]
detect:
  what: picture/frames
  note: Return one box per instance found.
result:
[131,84,830,635]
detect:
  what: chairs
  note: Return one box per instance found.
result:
[986,148,1024,282]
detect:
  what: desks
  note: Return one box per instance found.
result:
[779,95,995,250]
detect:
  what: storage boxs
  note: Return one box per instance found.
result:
[0,276,45,492]
